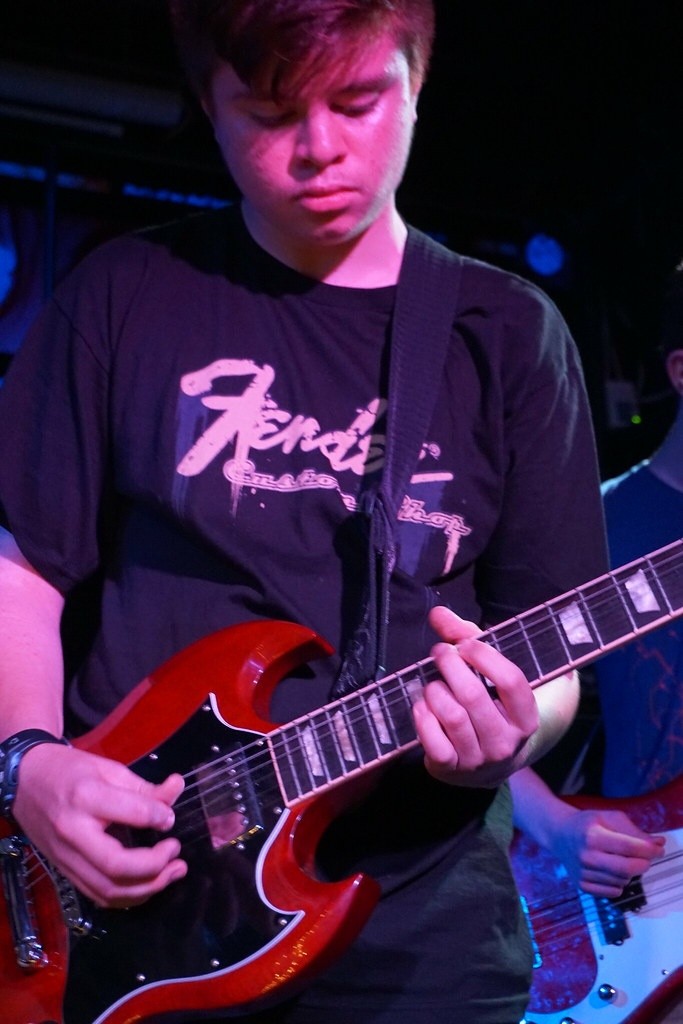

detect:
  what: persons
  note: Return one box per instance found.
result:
[505,267,682,1024]
[0,0,599,1024]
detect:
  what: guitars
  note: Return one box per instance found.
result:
[508,773,683,1024]
[0,537,682,1023]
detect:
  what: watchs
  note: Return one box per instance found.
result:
[0,728,74,825]
